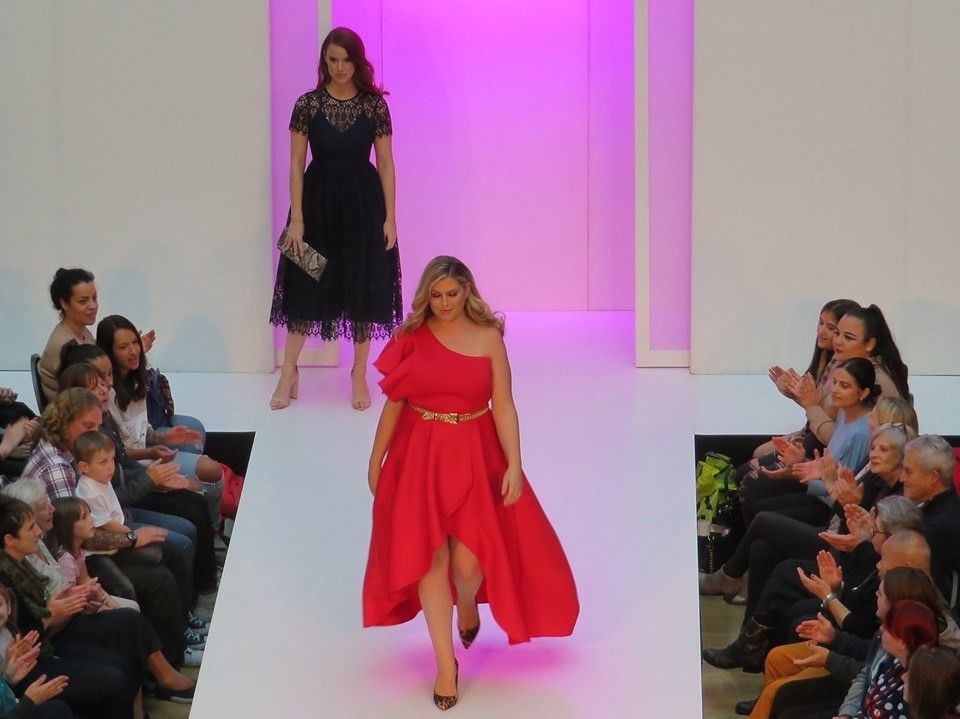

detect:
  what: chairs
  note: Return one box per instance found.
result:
[30,353,48,413]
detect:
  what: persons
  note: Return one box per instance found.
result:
[360,253,582,713]
[698,296,960,719]
[266,25,403,411]
[1,265,234,719]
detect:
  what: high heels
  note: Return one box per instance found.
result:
[351,368,372,412]
[432,657,459,710]
[268,363,300,411]
[456,597,480,649]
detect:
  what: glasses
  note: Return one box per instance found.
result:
[871,526,886,535]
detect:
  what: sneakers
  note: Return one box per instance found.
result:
[186,612,210,635]
[182,628,208,650]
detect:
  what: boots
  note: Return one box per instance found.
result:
[703,618,766,675]
[699,564,745,602]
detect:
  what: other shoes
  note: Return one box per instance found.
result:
[154,679,197,703]
[735,696,759,715]
[183,647,205,666]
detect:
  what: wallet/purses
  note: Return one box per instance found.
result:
[277,227,327,284]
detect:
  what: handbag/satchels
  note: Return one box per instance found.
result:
[698,520,730,574]
[713,468,742,526]
